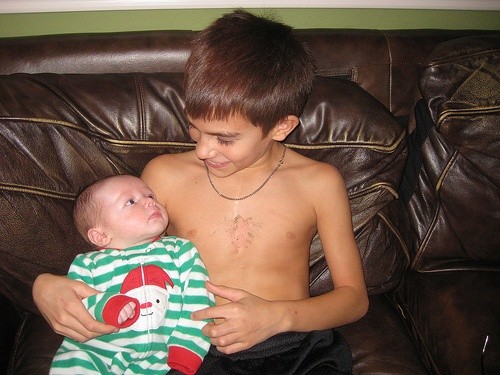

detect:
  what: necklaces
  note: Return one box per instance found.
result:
[204,142,287,201]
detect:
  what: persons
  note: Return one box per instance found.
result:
[48,174,218,375]
[32,7,370,375]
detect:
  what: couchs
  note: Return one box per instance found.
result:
[0,27,500,375]
